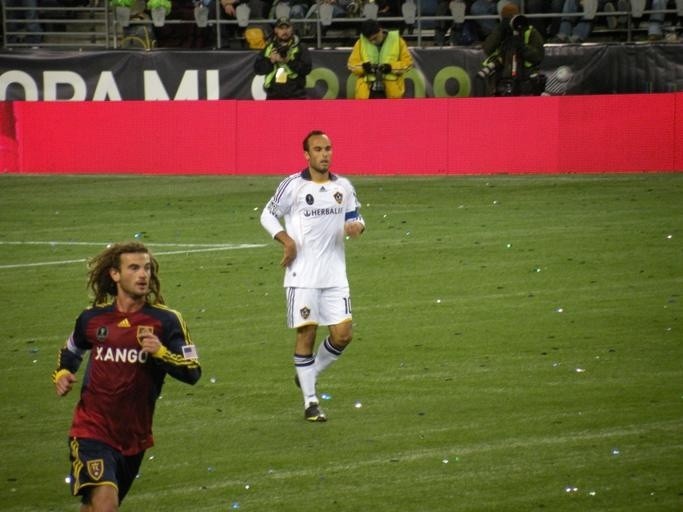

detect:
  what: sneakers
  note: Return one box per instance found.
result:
[305,402,326,422]
[294,373,301,389]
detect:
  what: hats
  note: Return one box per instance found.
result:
[275,16,291,27]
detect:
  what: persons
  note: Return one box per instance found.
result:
[251,17,311,99]
[0,0,44,43]
[54,242,202,508]
[346,19,415,98]
[260,131,366,423]
[481,3,546,96]
[150,0,683,48]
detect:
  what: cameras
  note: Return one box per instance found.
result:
[277,45,289,57]
[368,64,386,91]
[477,58,503,80]
